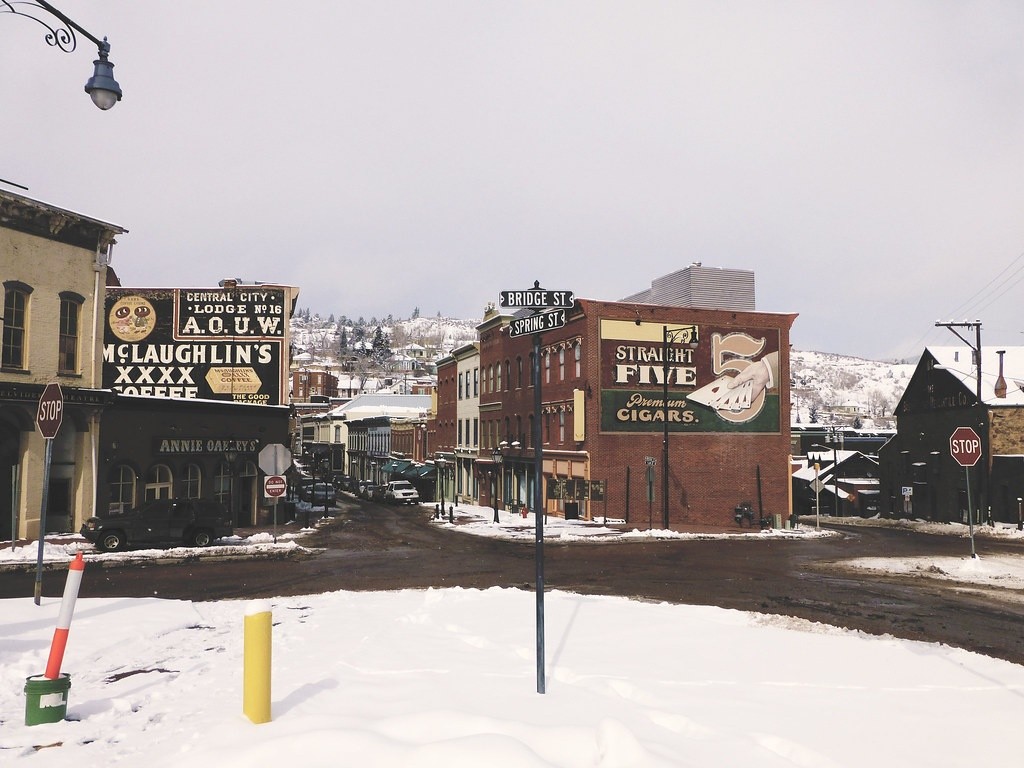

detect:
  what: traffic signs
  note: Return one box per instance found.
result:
[509,310,566,339]
[497,290,575,310]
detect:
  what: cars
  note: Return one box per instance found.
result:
[294,463,388,507]
[385,479,419,505]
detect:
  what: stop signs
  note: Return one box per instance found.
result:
[949,427,983,466]
[36,382,64,439]
[263,475,287,499]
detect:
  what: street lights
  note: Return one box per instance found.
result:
[934,363,984,526]
[811,443,840,517]
[434,454,447,515]
[490,444,503,523]
[661,324,699,528]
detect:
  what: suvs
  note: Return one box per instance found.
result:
[79,496,234,552]
